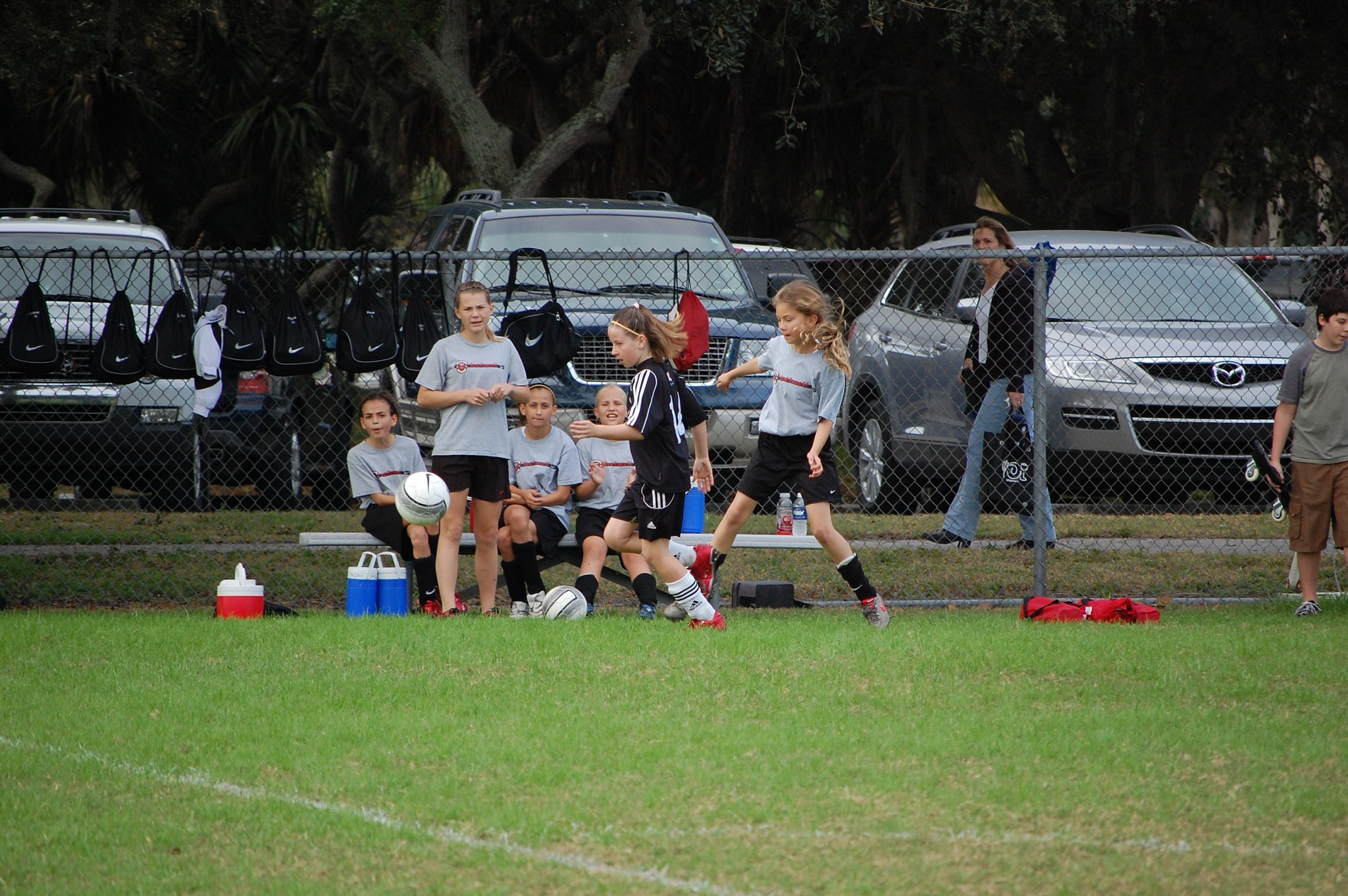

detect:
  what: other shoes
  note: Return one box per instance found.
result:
[442,608,460,616]
[485,608,501,618]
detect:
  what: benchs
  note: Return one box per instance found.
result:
[300,532,825,608]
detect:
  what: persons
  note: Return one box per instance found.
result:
[663,280,892,628]
[916,218,1057,551]
[497,383,583,618]
[570,303,726,629]
[346,392,468,615]
[1266,293,1348,616]
[574,384,657,620]
[414,281,530,617]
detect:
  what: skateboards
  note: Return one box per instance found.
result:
[1243,435,1289,524]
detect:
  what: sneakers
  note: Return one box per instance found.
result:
[664,600,687,622]
[586,603,593,615]
[689,611,726,631]
[422,599,439,618]
[861,594,889,628]
[526,590,546,618]
[1295,600,1322,617]
[637,604,654,620]
[433,586,468,614]
[508,601,528,619]
[686,545,720,600]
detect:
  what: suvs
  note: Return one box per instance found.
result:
[724,235,827,306]
[395,184,781,501]
[1,206,228,473]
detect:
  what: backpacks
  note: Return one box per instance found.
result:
[1,243,76,374]
[86,247,449,382]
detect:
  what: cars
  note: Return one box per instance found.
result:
[185,269,299,460]
[840,222,1311,506]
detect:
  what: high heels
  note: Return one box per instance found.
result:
[922,528,971,548]
[1007,537,1055,552]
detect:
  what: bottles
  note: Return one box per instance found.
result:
[792,492,807,536]
[776,493,793,535]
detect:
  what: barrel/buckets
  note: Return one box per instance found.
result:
[216,562,265,618]
[345,551,379,616]
[375,551,408,616]
[680,487,705,533]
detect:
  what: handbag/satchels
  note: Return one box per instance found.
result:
[666,290,711,373]
[498,301,580,378]
[1021,593,1161,624]
[986,406,1034,515]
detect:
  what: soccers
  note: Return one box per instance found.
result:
[542,585,587,619]
[395,472,450,525]
